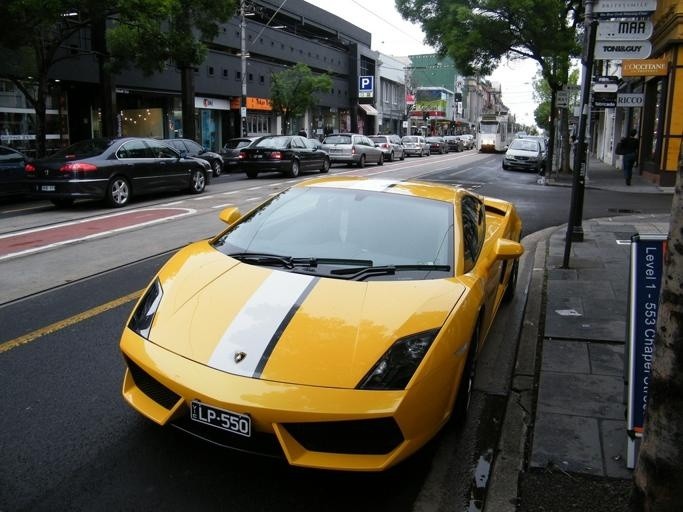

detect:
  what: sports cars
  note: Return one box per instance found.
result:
[118,174,525,475]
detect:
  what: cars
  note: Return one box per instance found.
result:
[0,145,38,202]
[321,133,383,168]
[368,134,407,162]
[442,136,464,153]
[459,134,474,150]
[239,135,331,178]
[424,136,448,154]
[158,138,225,176]
[25,136,214,206]
[401,135,431,157]
[221,137,262,169]
[502,134,549,172]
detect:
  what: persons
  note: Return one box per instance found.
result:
[619,129,640,186]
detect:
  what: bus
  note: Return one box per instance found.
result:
[475,110,515,153]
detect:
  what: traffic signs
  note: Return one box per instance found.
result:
[591,0,657,12]
[592,76,618,109]
[592,40,652,60]
[595,11,653,18]
[592,19,655,42]
[555,90,569,109]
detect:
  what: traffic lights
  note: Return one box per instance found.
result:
[454,93,462,102]
[422,111,430,121]
